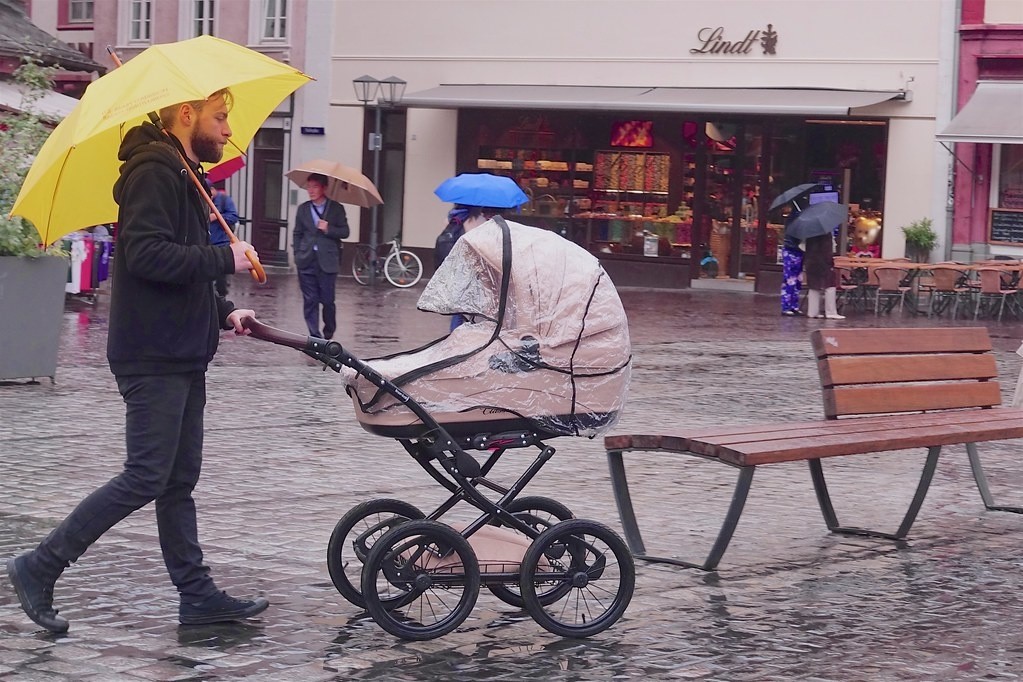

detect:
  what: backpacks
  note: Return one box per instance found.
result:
[434,215,466,270]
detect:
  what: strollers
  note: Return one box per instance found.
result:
[236,212,637,644]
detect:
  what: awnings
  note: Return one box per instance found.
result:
[393,83,905,116]
[934,80,1023,144]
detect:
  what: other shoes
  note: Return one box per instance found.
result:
[791,308,803,316]
[807,312,824,318]
[826,314,846,319]
[307,356,318,365]
[782,310,794,316]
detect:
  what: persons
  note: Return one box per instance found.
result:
[806,232,847,319]
[205,178,237,302]
[449,204,487,332]
[740,184,758,220]
[6,88,269,633]
[293,173,350,340]
[781,198,805,316]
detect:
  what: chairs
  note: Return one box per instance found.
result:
[798,254,1023,323]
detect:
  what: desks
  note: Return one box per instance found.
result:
[865,262,927,315]
[976,259,1023,264]
[970,266,1023,321]
[837,258,882,263]
[837,262,880,311]
[918,264,980,317]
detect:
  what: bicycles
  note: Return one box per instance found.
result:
[352,235,423,287]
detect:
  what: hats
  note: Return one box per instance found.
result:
[205,178,212,188]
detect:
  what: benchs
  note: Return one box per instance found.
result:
[606,325,1023,573]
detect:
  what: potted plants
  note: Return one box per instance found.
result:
[900,217,940,263]
[0,35,73,384]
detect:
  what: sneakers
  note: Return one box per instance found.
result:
[8,542,70,632]
[178,590,270,625]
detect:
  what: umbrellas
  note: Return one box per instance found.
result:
[9,34,317,284]
[204,156,246,184]
[283,159,384,230]
[768,183,820,212]
[784,201,848,240]
[433,174,530,208]
